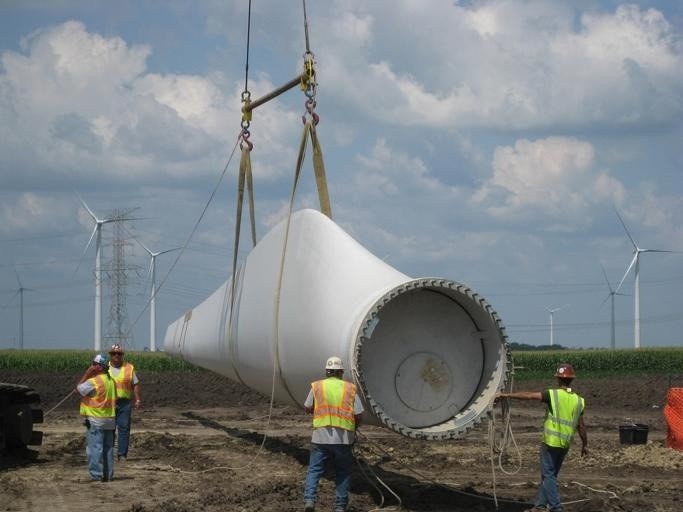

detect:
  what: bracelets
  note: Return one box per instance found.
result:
[135,399,142,403]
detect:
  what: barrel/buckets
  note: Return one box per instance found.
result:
[619,424,635,444]
[635,422,650,444]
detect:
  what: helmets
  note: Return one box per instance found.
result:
[109,344,125,355]
[325,356,345,370]
[92,354,111,368]
[553,363,577,379]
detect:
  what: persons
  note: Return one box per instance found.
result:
[494,361,590,512]
[303,355,365,512]
[105,343,143,462]
[75,352,118,482]
